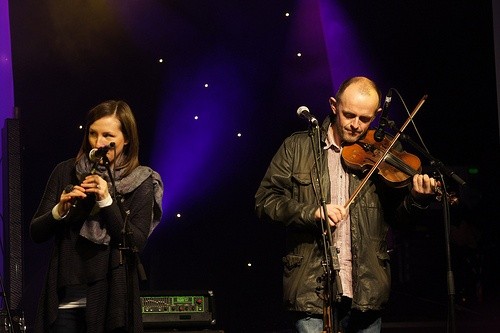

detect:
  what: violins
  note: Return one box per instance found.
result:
[341,128,460,207]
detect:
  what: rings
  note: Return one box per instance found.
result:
[64,184,74,193]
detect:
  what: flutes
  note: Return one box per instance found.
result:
[57,158,100,231]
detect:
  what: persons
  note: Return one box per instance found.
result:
[254,76,442,333]
[28,101,164,333]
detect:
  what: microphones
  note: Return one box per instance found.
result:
[88,142,115,161]
[373,88,393,142]
[297,106,318,125]
